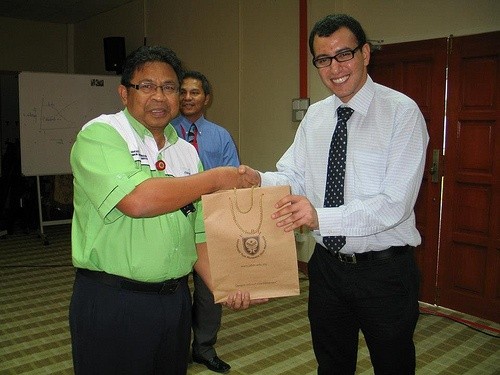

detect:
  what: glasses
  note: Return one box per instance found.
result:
[312,44,360,68]
[128,82,179,96]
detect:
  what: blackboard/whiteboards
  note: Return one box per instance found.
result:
[18,70,124,176]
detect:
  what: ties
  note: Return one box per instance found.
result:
[323,105,354,256]
[188,123,199,145]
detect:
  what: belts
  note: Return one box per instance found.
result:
[317,245,406,264]
[76,268,189,291]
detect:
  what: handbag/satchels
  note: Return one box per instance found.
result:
[201,184,300,302]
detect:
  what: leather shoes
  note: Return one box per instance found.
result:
[192,349,231,372]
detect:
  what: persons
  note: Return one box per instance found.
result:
[170,71,242,373]
[237,13,432,375]
[68,41,260,375]
